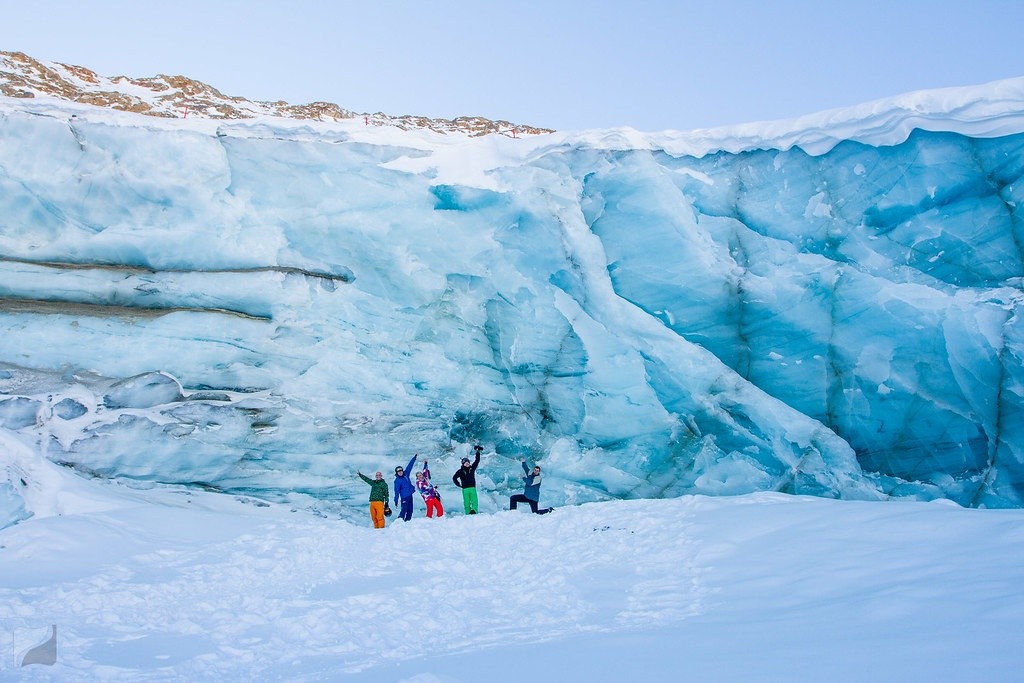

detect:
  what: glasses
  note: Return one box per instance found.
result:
[397,470,403,472]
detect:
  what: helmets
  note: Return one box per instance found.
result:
[384,508,392,517]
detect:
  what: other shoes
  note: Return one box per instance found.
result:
[548,507,553,513]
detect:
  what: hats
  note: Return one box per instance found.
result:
[462,458,470,464]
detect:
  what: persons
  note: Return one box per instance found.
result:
[394,454,418,522]
[415,458,443,519]
[452,449,481,516]
[509,458,553,516]
[356,470,391,529]
